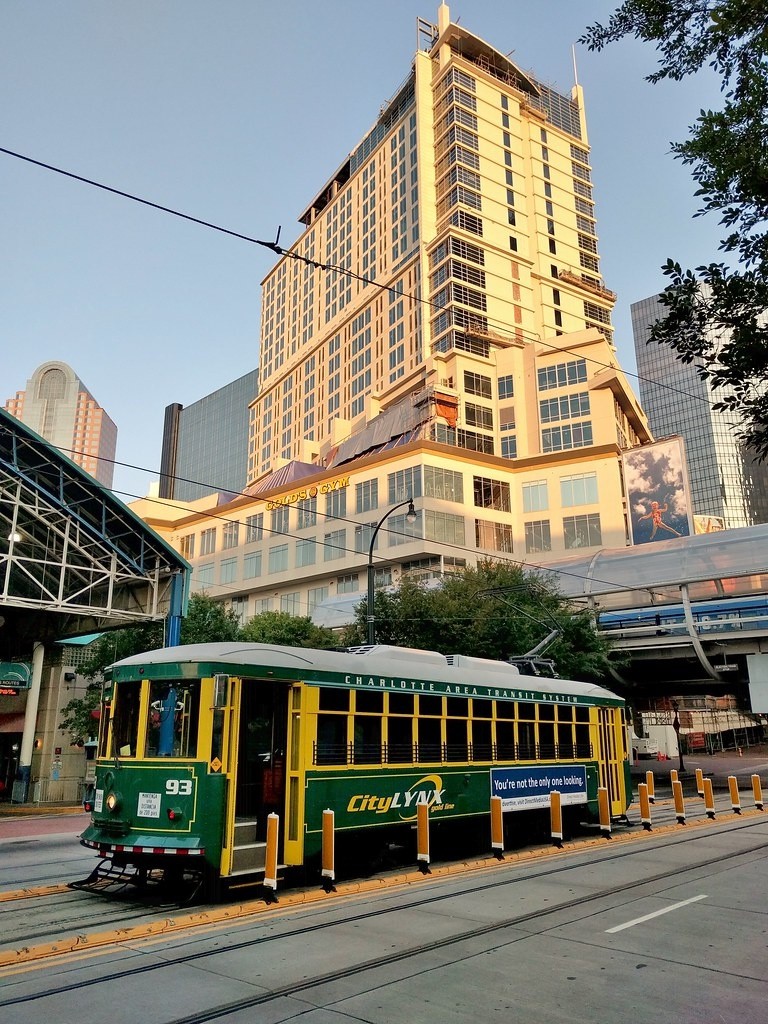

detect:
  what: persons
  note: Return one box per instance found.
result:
[0,778,5,802]
[638,502,682,542]
[148,719,161,753]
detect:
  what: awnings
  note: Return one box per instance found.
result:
[606,629,768,655]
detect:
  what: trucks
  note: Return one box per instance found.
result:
[632,737,658,758]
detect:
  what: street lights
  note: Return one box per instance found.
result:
[365,498,416,645]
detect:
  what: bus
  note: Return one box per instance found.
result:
[64,642,634,911]
